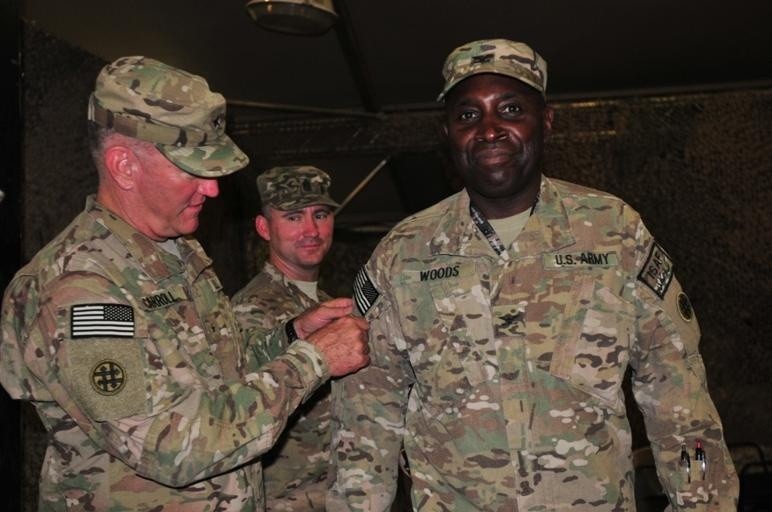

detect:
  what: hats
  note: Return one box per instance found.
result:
[86,55,252,179]
[434,38,550,104]
[256,164,342,212]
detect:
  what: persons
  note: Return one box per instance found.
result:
[324,38,740,512]
[234,164,342,512]
[1,55,370,512]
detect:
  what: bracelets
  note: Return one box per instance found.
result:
[286,317,298,344]
